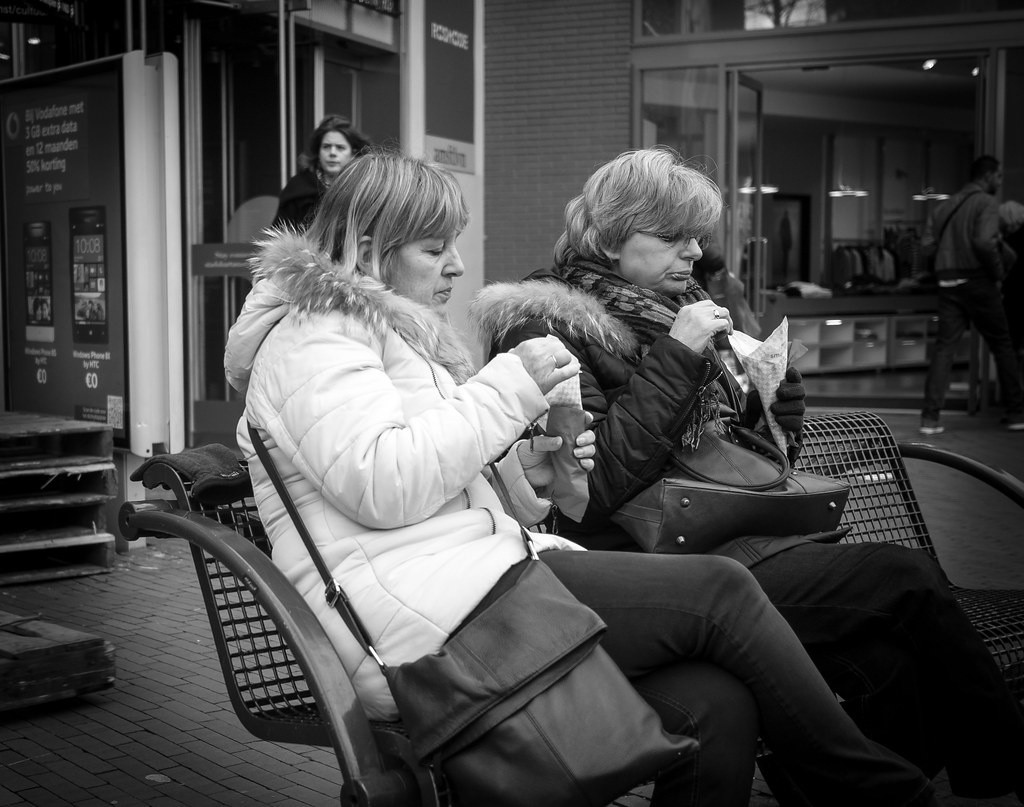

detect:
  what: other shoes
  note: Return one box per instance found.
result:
[1006,413,1024,430]
[919,417,944,434]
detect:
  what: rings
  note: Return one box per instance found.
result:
[552,356,558,367]
[715,310,719,317]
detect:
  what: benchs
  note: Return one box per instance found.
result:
[115,407,1024,807]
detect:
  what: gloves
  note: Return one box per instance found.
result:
[769,367,806,431]
[130,443,254,506]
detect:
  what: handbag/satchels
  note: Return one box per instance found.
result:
[382,558,701,807]
[610,416,850,554]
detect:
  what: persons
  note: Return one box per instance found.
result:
[918,154,1024,436]
[270,114,373,237]
[76,300,104,321]
[32,298,50,321]
[222,151,934,807]
[473,145,1024,807]
